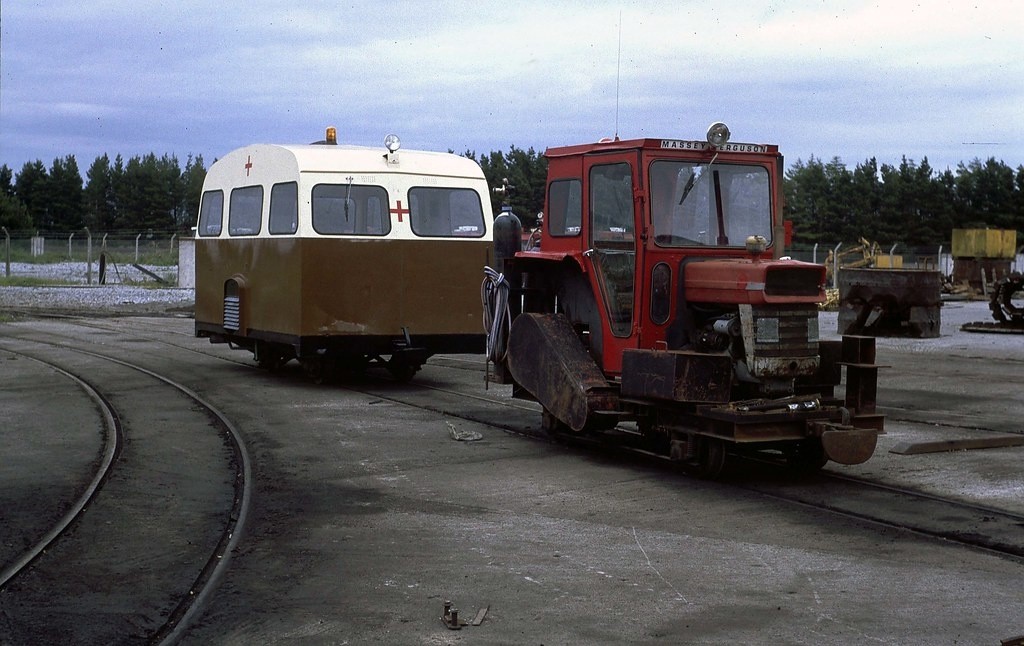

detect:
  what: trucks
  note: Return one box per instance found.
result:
[478,121,891,483]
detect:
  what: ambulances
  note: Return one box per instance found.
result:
[190,126,496,384]
